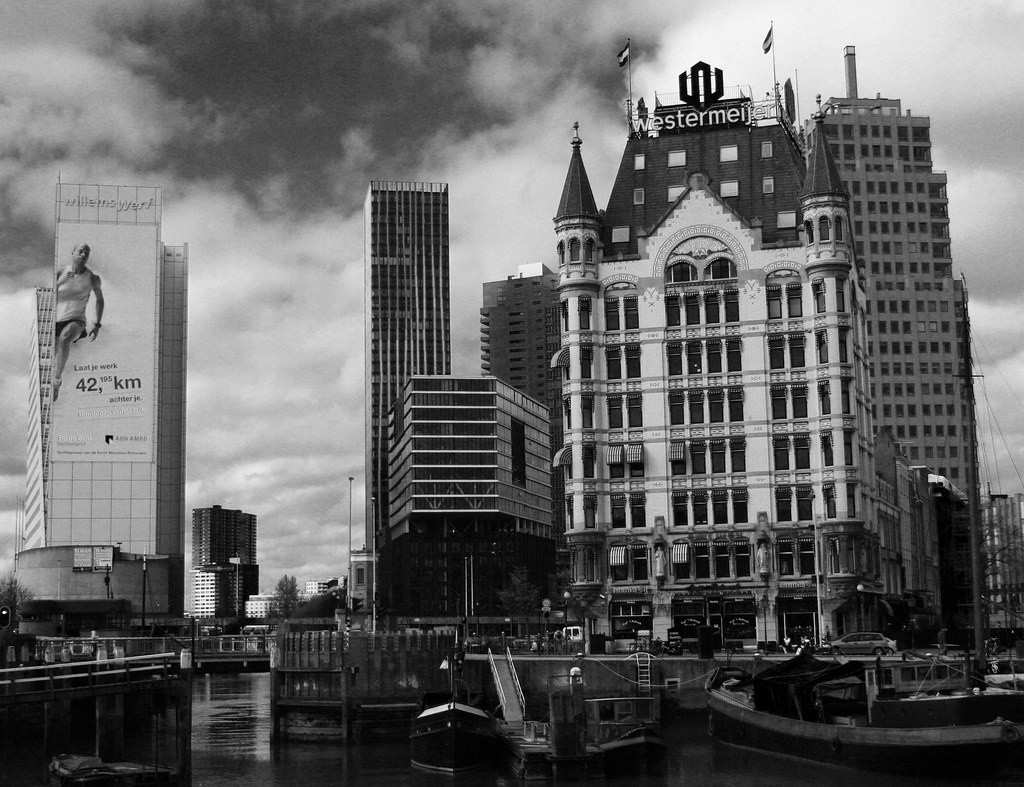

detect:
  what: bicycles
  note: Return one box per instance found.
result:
[632,637,662,658]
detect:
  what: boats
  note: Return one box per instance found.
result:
[703,640,1024,778]
[584,697,671,766]
[49,754,170,787]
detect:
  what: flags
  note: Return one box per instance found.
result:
[617,42,631,67]
[762,27,774,53]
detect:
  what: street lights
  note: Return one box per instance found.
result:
[644,592,654,654]
[410,700,499,773]
[563,591,570,656]
[720,591,724,648]
[907,597,916,649]
[348,476,354,612]
[475,600,480,636]
[759,594,769,656]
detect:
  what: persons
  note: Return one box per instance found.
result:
[938,628,947,654]
[53,243,105,403]
[757,543,770,572]
[654,547,668,575]
[249,629,255,635]
[534,634,543,651]
[783,636,792,654]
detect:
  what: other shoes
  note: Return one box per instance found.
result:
[53,376,62,402]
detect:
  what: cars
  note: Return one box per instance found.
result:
[860,635,898,656]
[821,631,889,656]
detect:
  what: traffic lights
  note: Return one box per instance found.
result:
[0,607,12,628]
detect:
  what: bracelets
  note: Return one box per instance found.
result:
[95,323,102,328]
